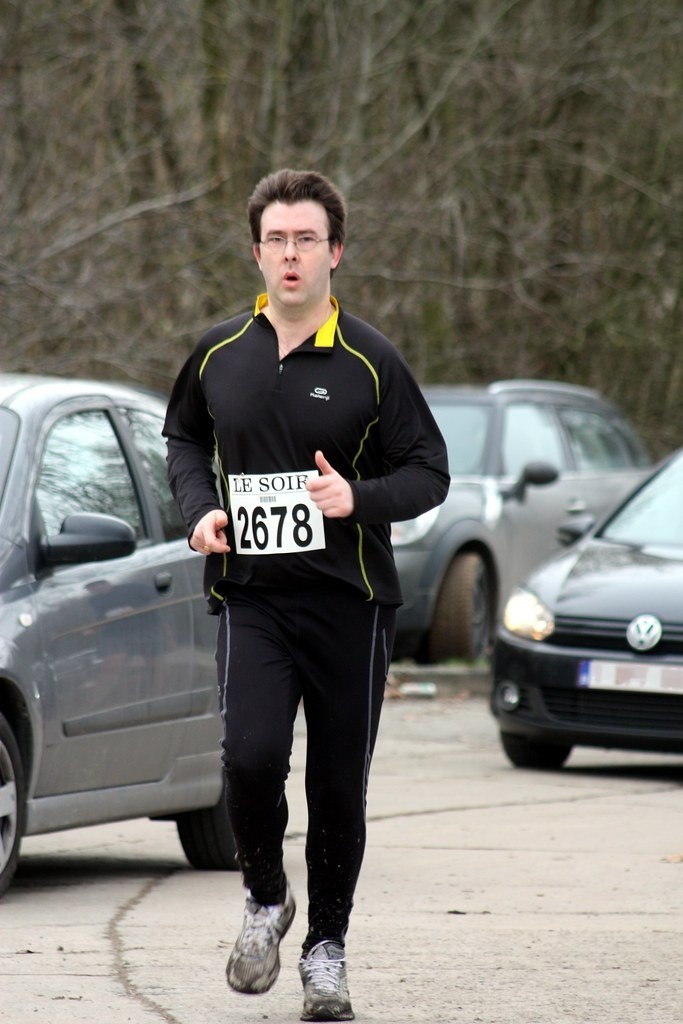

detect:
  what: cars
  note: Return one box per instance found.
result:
[0,370,250,897]
[486,448,683,777]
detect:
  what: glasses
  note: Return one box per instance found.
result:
[259,235,328,251]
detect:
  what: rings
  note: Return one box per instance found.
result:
[204,546,209,551]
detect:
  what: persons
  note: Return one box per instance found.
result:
[162,169,451,1022]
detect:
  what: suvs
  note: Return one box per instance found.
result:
[382,377,665,668]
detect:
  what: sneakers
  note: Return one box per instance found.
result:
[297,939,355,1021]
[224,874,297,995]
